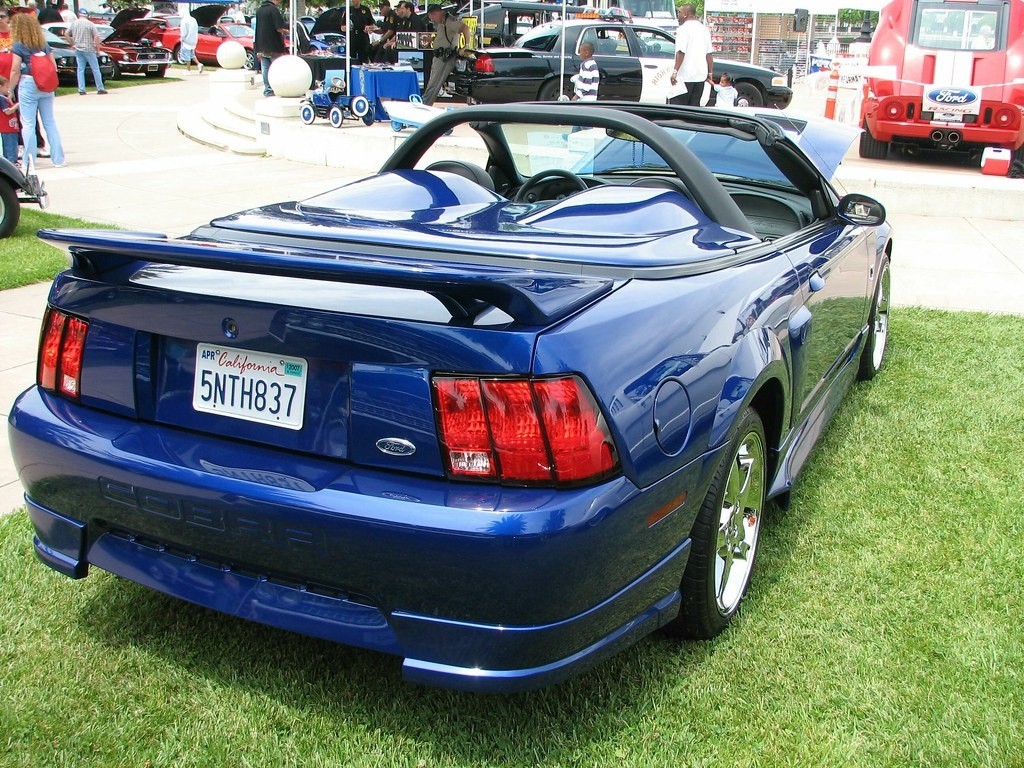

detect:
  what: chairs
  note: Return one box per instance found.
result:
[629,175,704,213]
[425,159,496,193]
[208,26,217,35]
[602,38,618,55]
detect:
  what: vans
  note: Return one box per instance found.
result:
[417,0,605,52]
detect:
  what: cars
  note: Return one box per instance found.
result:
[441,6,795,111]
[0,0,357,86]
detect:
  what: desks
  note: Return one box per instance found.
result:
[296,57,360,90]
[347,66,420,122]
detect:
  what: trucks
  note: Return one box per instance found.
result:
[855,1,1024,177]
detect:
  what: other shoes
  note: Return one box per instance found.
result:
[199,64,204,73]
[37,147,51,158]
[267,91,274,96]
[16,145,25,160]
[98,90,108,94]
[186,71,192,76]
[53,159,67,168]
[79,92,86,95]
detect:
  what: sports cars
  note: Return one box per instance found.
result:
[4,94,899,700]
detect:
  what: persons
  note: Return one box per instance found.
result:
[970,25,992,49]
[707,72,738,107]
[309,6,346,37]
[251,17,261,74]
[569,42,600,133]
[66,8,108,94]
[365,0,426,64]
[178,7,204,76]
[100,2,138,14]
[255,0,292,98]
[421,3,470,106]
[0,7,53,160]
[7,12,68,170]
[228,4,247,25]
[24,0,78,36]
[349,0,376,64]
[0,75,22,168]
[665,4,714,106]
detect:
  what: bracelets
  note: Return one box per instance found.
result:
[673,70,677,74]
[465,43,469,45]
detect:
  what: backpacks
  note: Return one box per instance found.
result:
[29,49,59,92]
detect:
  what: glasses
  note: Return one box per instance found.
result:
[0,15,9,19]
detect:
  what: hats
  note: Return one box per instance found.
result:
[394,1,406,7]
[377,0,390,7]
[78,8,87,14]
